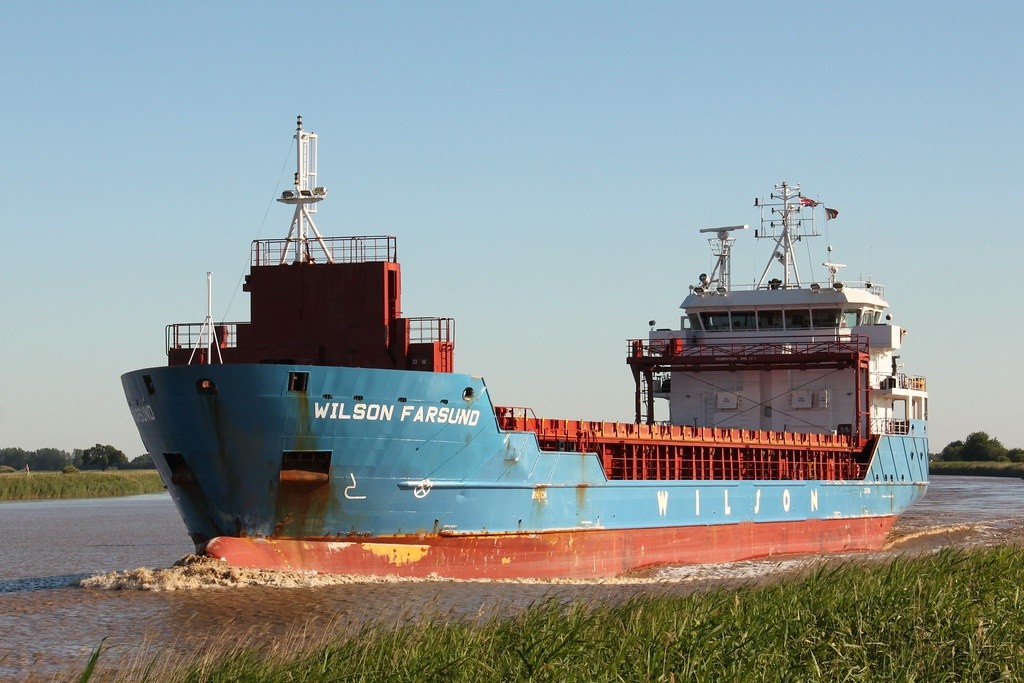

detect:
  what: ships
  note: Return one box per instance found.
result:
[118,114,930,584]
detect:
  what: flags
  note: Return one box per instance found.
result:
[801,197,817,208]
[825,208,838,220]
